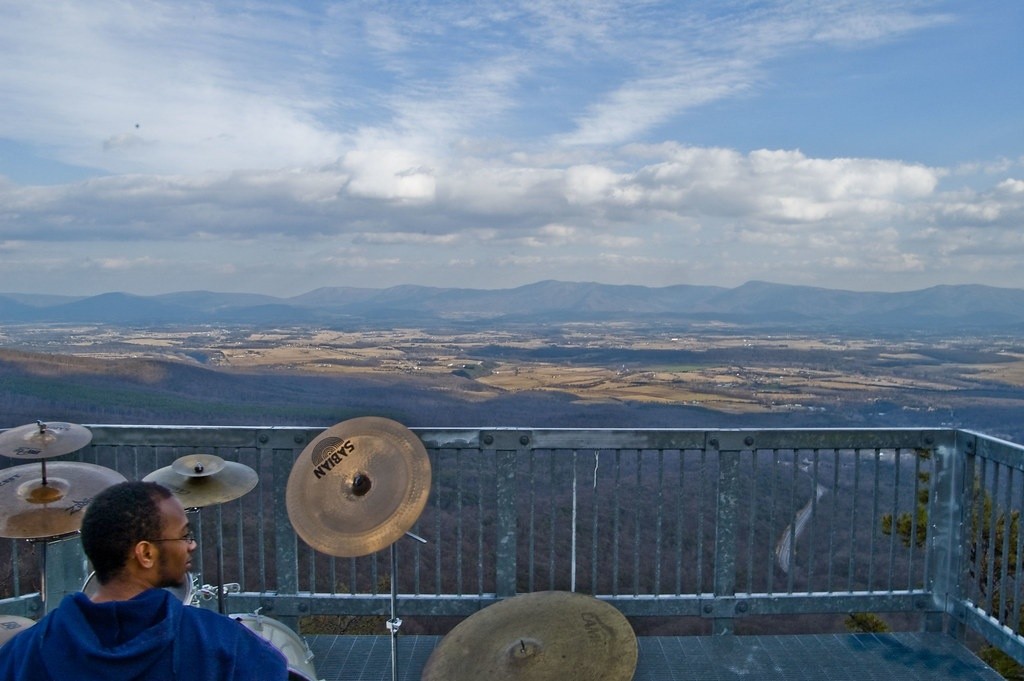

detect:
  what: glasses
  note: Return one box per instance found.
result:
[150,530,195,545]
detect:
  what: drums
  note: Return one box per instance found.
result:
[80,569,200,611]
[221,612,318,681]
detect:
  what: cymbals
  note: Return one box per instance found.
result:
[142,459,259,507]
[172,453,226,478]
[0,461,129,539]
[285,414,433,558]
[0,420,94,459]
[0,614,37,649]
[419,589,639,681]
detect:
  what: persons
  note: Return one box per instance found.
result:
[0,481,288,681]
